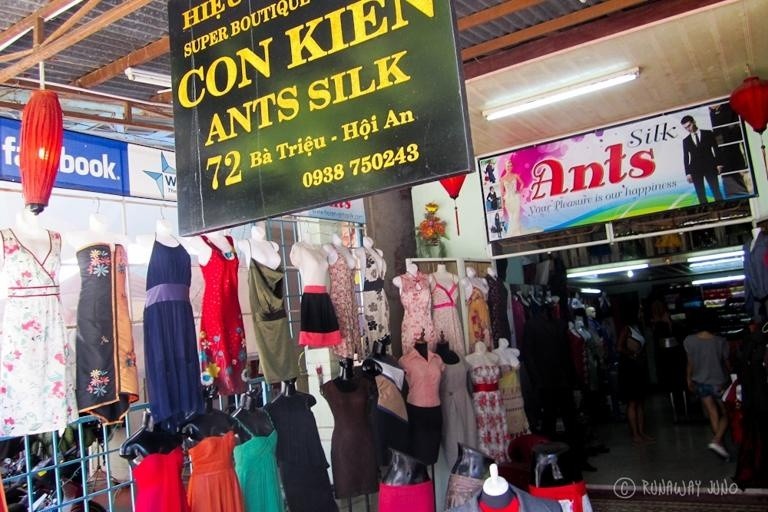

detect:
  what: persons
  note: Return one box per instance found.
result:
[641,291,686,421]
[487,186,499,212]
[500,160,544,237]
[495,213,503,237]
[683,307,731,462]
[681,115,724,204]
[484,160,496,183]
[616,304,656,448]
[1,219,619,512]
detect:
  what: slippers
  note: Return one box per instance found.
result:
[640,431,656,441]
[631,442,647,449]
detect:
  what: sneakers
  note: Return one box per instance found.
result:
[707,442,728,460]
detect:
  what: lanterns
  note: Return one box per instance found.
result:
[440,173,466,236]
[20,88,63,213]
[730,76,768,178]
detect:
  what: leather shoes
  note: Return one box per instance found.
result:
[581,462,598,473]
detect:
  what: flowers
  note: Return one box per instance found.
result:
[414,202,451,245]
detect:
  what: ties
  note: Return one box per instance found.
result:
[694,133,699,145]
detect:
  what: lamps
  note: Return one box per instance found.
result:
[482,67,641,122]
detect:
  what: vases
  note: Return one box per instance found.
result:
[427,245,442,257]
